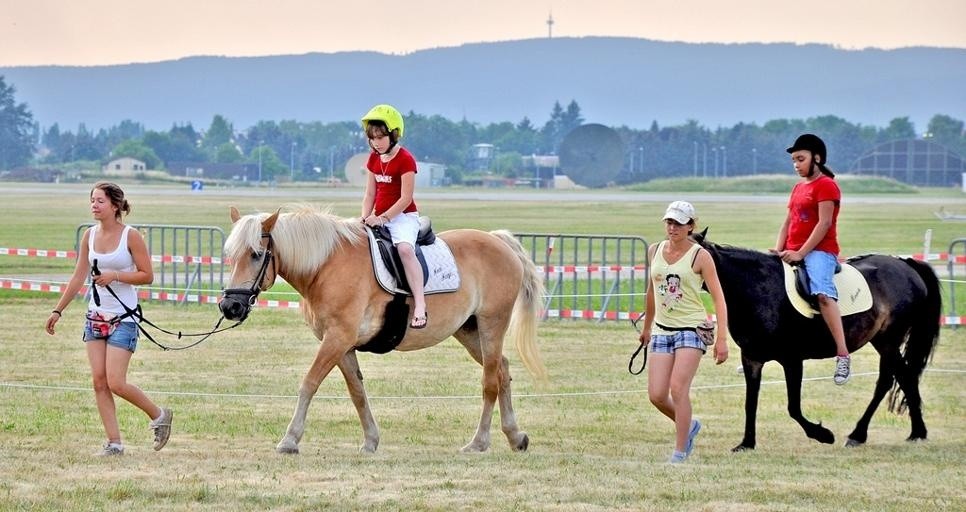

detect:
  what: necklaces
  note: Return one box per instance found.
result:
[379,155,391,178]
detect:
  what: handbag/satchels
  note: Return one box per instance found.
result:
[85,306,139,338]
[654,322,715,344]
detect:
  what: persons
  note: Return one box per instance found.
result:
[638,200,728,460]
[736,133,852,385]
[45,181,173,457]
[358,105,429,331]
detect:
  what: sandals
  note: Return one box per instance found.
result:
[410,311,428,329]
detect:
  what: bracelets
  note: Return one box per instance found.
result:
[378,216,384,228]
[52,310,62,317]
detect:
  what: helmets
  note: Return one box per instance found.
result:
[360,105,404,138]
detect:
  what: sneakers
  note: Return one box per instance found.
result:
[688,420,700,437]
[94,444,124,457]
[834,356,851,384]
[149,407,174,450]
[671,438,693,461]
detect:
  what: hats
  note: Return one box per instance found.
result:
[662,201,698,228]
[786,133,826,164]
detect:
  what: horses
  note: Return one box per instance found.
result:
[690,225,943,452]
[217,205,544,455]
